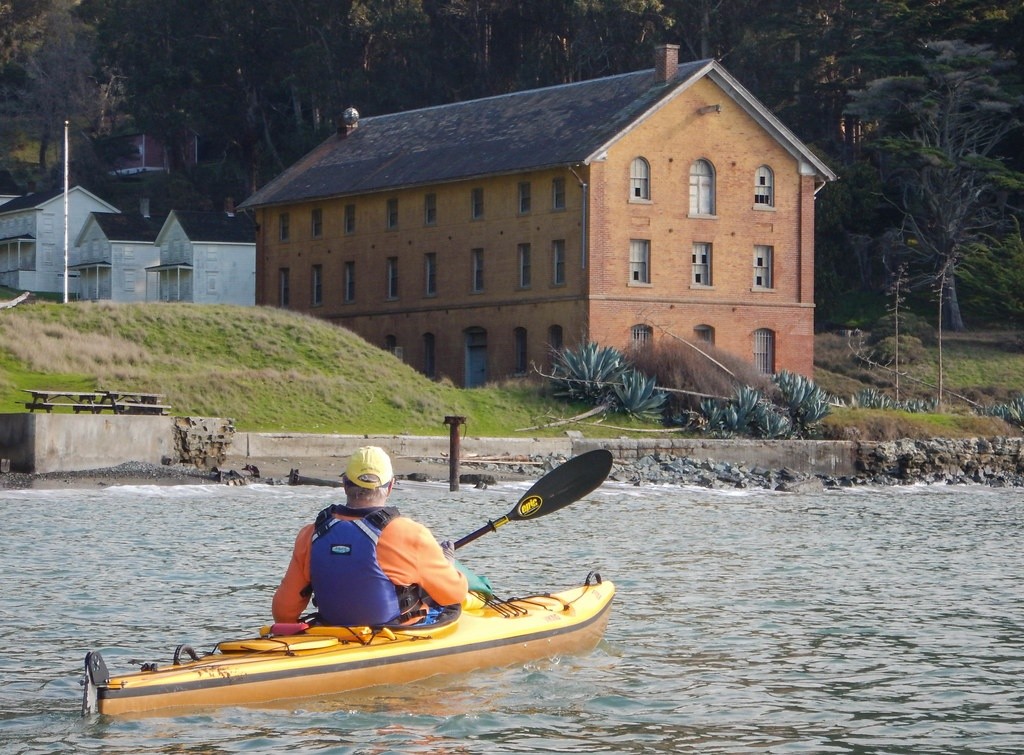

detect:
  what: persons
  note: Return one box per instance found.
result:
[271,445,469,624]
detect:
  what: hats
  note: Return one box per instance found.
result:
[338,445,395,490]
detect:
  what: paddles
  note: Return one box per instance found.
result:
[450,448,613,551]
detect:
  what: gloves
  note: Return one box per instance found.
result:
[440,539,455,562]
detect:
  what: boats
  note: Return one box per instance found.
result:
[83,570,614,728]
